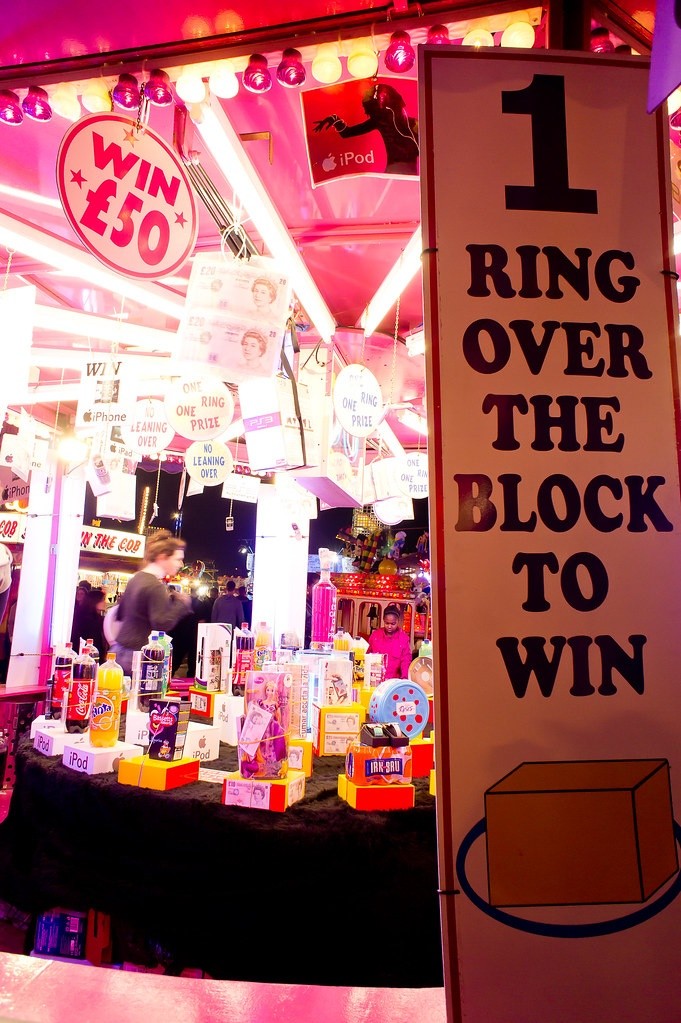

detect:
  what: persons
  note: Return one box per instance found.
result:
[107,530,192,676]
[366,606,412,684]
[70,579,107,655]
[211,581,244,629]
[304,572,320,649]
[166,586,253,678]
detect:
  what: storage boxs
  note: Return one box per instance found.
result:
[33,727,416,815]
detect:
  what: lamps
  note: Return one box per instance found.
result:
[183,81,336,345]
[358,224,423,338]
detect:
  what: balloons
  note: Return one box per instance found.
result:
[378,559,397,575]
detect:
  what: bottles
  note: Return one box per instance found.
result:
[89,652,124,747]
[157,631,170,700]
[332,626,344,649]
[231,622,255,697]
[311,569,337,642]
[50,642,78,720]
[253,621,270,671]
[65,647,96,734]
[351,636,364,684]
[137,635,165,713]
[418,638,432,658]
[85,639,99,696]
